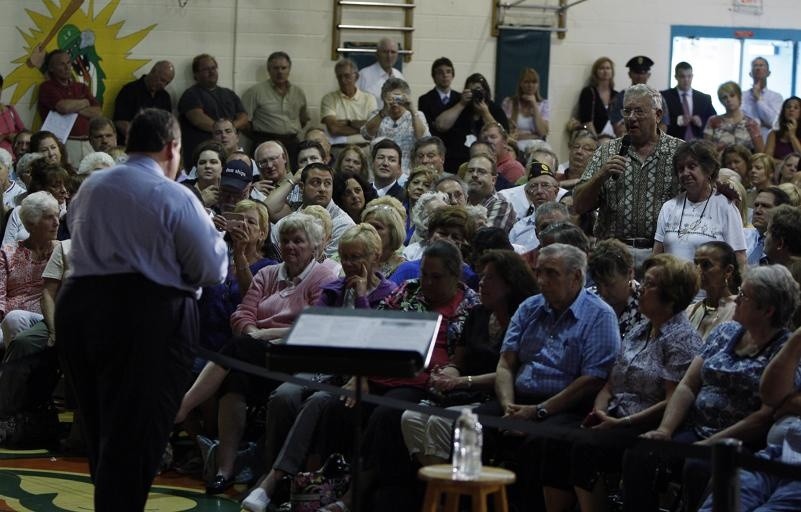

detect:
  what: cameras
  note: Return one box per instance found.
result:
[393,94,404,104]
[471,89,483,101]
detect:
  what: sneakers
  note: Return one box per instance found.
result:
[1,414,33,450]
[205,470,237,496]
[239,487,272,512]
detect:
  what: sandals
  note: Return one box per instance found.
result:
[315,500,351,512]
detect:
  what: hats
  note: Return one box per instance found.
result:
[527,162,556,182]
[220,158,253,192]
[626,55,654,74]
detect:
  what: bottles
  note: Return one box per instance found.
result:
[454,408,484,479]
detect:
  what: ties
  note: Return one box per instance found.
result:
[680,93,695,142]
[442,96,449,105]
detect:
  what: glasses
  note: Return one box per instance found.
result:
[619,105,657,120]
[735,285,768,309]
[466,168,496,177]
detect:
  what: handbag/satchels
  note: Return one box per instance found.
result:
[286,450,354,512]
[565,85,598,142]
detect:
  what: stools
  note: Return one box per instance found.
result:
[415,461,520,510]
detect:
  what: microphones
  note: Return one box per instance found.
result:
[612,134,631,181]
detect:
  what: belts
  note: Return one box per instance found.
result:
[254,132,299,142]
[621,235,654,252]
[331,142,369,150]
[67,136,91,141]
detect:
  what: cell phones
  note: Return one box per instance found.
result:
[222,212,246,233]
